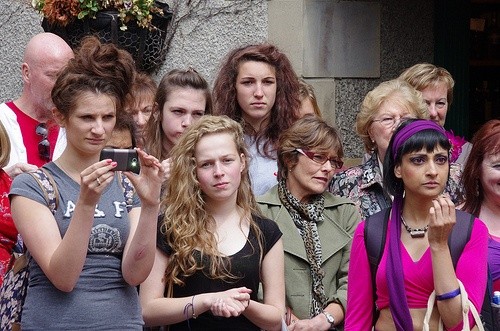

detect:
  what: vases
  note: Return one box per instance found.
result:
[40,13,173,74]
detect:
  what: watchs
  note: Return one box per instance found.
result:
[319,310,335,328]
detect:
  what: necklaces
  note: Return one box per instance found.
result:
[400,216,428,237]
[57,159,81,185]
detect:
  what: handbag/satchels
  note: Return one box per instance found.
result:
[0,167,60,331]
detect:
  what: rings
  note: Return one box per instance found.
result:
[97,178,101,185]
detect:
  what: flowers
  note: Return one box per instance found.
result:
[32,0,164,27]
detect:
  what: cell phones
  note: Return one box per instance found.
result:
[100,148,138,171]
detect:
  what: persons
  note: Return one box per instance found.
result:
[138,114,285,331]
[9,35,165,331]
[455,119,500,331]
[0,32,474,219]
[254,113,361,331]
[0,121,20,287]
[344,118,488,331]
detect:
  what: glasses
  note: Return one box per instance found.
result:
[35,122,51,159]
[371,113,418,128]
[292,148,344,169]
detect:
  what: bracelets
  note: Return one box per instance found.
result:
[184,296,197,319]
[436,287,461,301]
